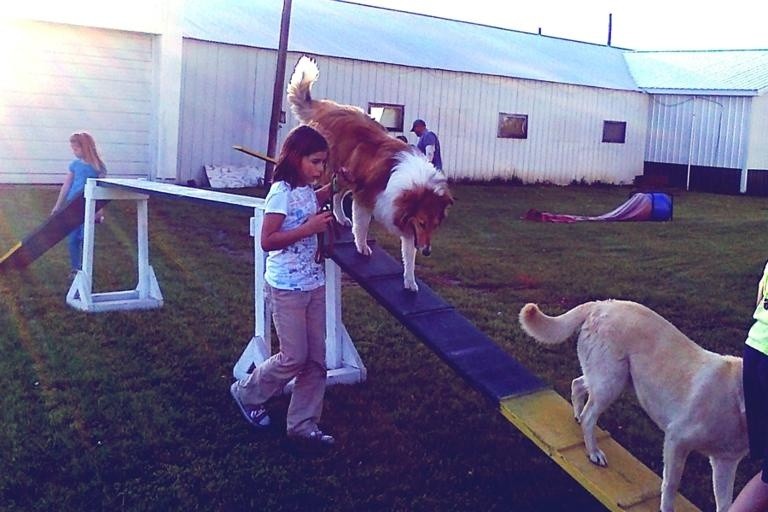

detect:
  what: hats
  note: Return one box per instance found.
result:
[409,119,425,132]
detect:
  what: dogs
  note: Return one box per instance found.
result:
[286,54,455,292]
[520,299,750,512]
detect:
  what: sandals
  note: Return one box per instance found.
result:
[287,426,335,443]
[230,381,272,429]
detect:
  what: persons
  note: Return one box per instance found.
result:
[722,257,767,512]
[408,117,442,174]
[46,130,109,279]
[229,124,337,449]
[396,134,407,143]
